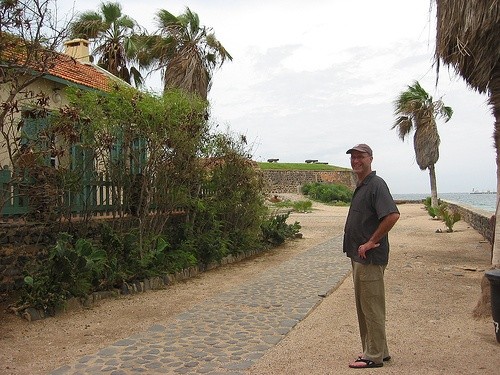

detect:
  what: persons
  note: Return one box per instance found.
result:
[343,144,400,367]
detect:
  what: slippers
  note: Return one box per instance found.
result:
[348,358,383,368]
[355,354,391,362]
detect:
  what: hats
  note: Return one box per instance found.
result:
[346,144,373,156]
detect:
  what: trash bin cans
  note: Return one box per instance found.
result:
[485,269,500,344]
